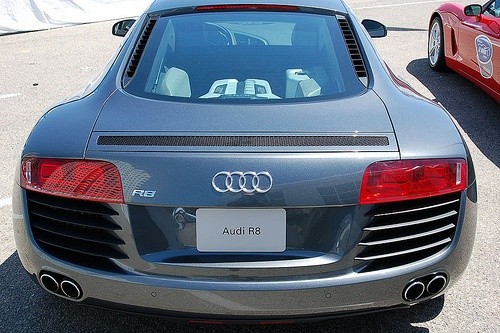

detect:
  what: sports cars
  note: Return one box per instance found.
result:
[14,0,479,320]
[428,0,500,103]
